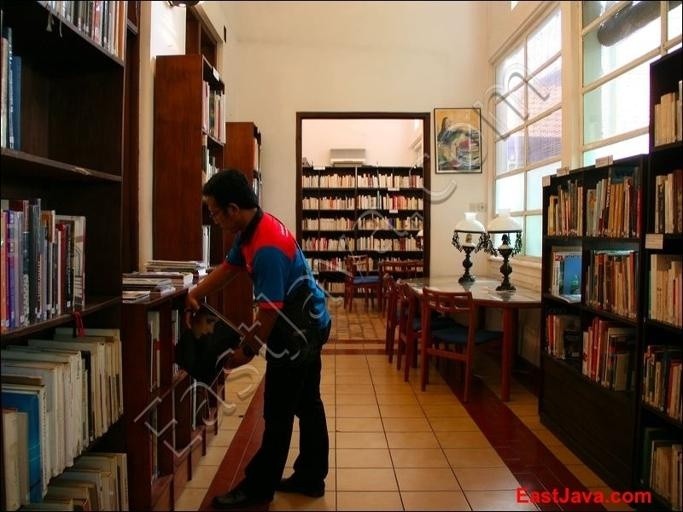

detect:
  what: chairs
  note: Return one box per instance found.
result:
[318,255,540,404]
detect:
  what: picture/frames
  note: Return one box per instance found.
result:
[434,107,484,175]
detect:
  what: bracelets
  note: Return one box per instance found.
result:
[242,345,259,358]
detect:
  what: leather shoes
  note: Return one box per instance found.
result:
[213,484,274,510]
[276,471,325,498]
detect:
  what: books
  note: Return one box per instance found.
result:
[174,303,246,387]
[253,137,263,208]
[544,80,683,512]
[302,172,424,293]
[0,0,226,511]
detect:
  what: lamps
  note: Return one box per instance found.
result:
[450,210,490,285]
[485,211,525,294]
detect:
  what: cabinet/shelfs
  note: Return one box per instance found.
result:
[641,45,683,512]
[534,152,646,512]
[0,0,262,511]
[301,163,423,307]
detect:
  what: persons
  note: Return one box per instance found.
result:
[188,170,331,510]
